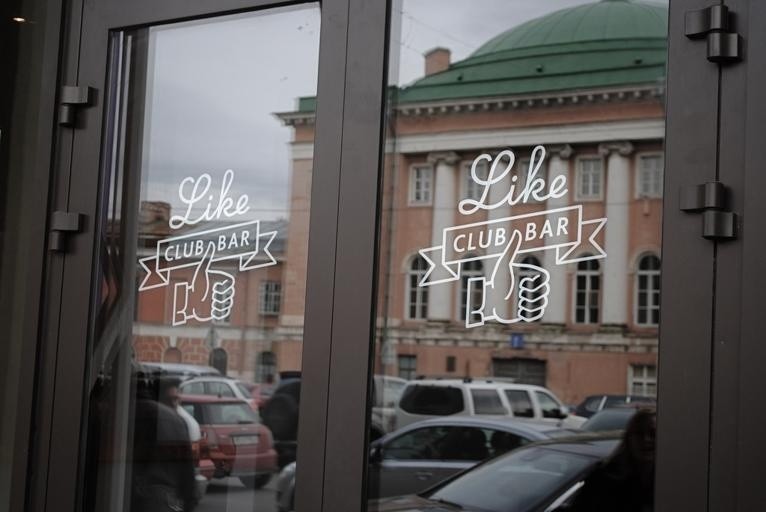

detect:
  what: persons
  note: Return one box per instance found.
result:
[462,430,489,460]
[152,379,201,467]
[130,379,194,512]
[568,407,657,512]
[422,427,448,459]
